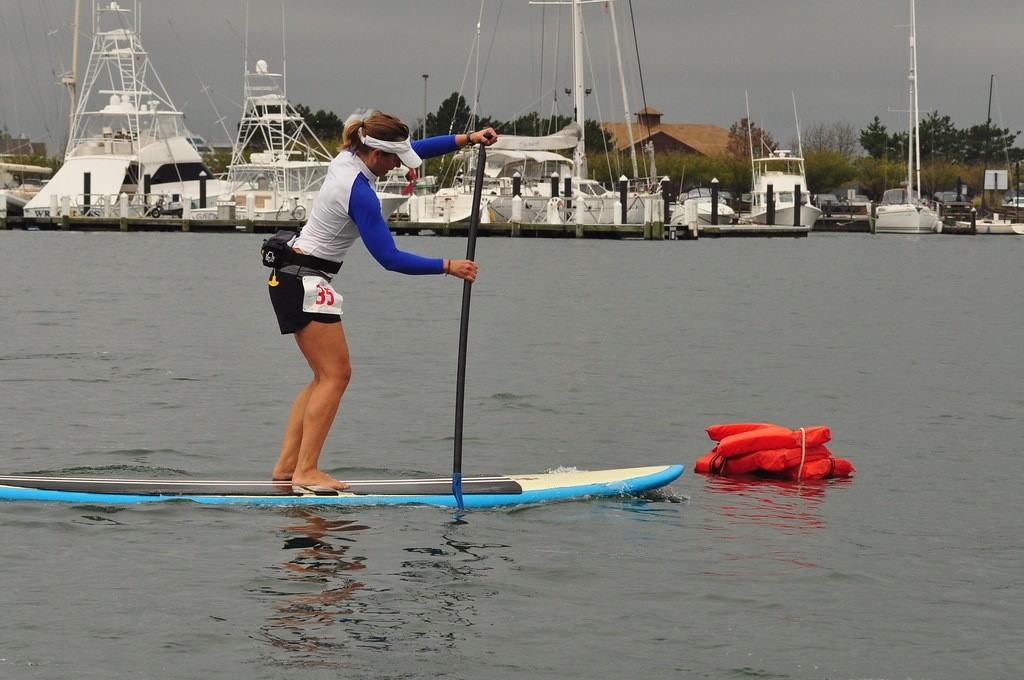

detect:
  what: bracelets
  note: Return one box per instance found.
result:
[446,258,452,276]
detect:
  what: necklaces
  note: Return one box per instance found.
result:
[265,106,499,489]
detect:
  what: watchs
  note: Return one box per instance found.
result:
[466,130,476,145]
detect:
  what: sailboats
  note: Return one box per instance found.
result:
[956,72,1024,236]
[871,0,942,235]
[402,0,665,239]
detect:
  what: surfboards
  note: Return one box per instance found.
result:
[1,463,687,503]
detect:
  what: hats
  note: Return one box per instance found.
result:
[358,126,422,169]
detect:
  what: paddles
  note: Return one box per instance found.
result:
[450,132,490,509]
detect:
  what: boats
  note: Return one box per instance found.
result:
[742,86,822,233]
[0,1,407,227]
[673,186,735,226]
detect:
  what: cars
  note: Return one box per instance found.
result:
[1005,195,1024,208]
[844,194,873,213]
[934,190,973,214]
[812,192,842,207]
